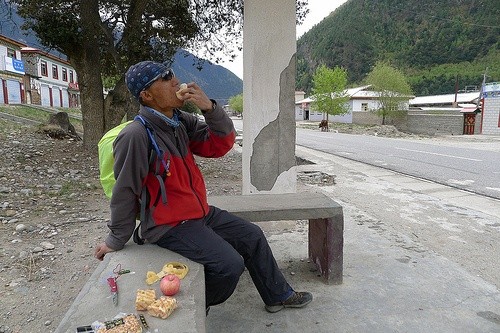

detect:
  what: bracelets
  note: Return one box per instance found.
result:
[212,102,216,109]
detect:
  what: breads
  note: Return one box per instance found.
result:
[147,295,178,319]
[135,289,156,311]
[97,314,143,333]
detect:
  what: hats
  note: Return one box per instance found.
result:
[125,61,170,98]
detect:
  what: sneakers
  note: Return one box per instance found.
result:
[265,290,313,313]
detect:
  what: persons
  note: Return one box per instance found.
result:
[94,61,313,316]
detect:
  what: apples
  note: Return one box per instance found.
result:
[160,274,180,296]
[176,83,190,100]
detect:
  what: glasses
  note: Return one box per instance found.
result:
[161,68,176,81]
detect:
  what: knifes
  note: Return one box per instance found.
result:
[108,278,118,305]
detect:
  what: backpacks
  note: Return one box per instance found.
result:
[96,115,169,245]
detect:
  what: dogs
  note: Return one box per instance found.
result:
[319,120,329,132]
